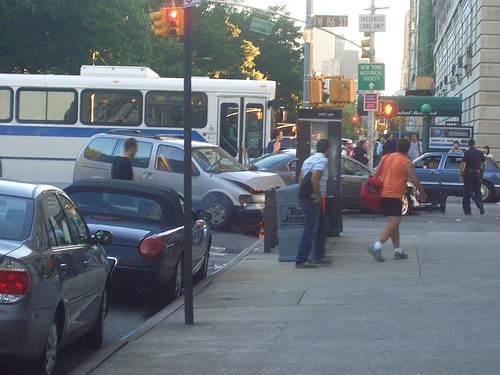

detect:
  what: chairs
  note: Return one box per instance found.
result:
[138,198,156,217]
[0,209,25,240]
[77,192,107,212]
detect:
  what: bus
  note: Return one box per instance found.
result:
[0,63,279,224]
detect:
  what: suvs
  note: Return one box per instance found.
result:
[72,127,289,232]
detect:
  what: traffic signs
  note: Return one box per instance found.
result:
[357,62,385,91]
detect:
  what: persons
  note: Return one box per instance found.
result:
[427,160,439,169]
[45,205,112,289]
[296,139,332,268]
[342,139,369,171]
[369,137,426,262]
[449,139,489,214]
[112,138,138,207]
[268,129,283,154]
[373,135,423,168]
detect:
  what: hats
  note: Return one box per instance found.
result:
[347,140,352,143]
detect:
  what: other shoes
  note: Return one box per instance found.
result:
[393,250,407,259]
[368,245,385,261]
[295,262,316,268]
[480,207,484,214]
[311,257,331,264]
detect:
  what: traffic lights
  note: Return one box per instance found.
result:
[361,31,370,58]
[149,8,167,37]
[351,115,359,123]
[162,7,183,37]
[383,101,397,115]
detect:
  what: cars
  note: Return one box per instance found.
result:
[0,178,121,375]
[410,150,500,204]
[248,146,414,217]
[59,177,213,303]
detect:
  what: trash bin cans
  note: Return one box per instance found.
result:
[275,183,315,261]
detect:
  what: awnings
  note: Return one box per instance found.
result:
[357,95,462,118]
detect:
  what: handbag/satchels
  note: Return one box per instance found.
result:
[360,176,382,209]
[299,171,313,196]
